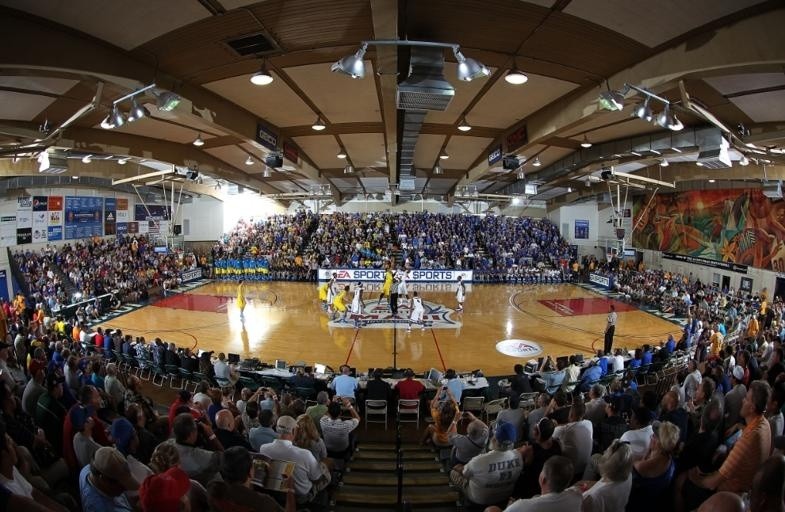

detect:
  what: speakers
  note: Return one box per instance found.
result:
[266,156,282,168]
[174,225,181,235]
[186,170,198,180]
[503,159,519,170]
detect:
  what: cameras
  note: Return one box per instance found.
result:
[261,388,267,391]
[443,387,448,390]
[337,398,342,402]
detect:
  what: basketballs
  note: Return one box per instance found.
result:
[391,245,397,253]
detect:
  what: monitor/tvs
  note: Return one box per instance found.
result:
[314,363,326,374]
[349,368,357,378]
[622,249,636,256]
[228,353,240,366]
[557,356,568,365]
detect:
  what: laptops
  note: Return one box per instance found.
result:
[576,354,586,363]
[276,360,289,372]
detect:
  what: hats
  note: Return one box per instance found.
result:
[95,447,140,490]
[494,423,518,442]
[29,359,45,371]
[0,342,13,350]
[278,416,300,430]
[139,467,191,511]
[733,366,744,380]
[652,420,662,444]
[47,374,65,390]
[604,395,625,410]
[70,403,96,428]
[111,418,133,443]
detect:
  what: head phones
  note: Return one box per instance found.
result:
[444,369,457,379]
[340,365,352,375]
[373,369,385,378]
[404,368,415,377]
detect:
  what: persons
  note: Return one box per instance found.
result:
[423,319,785,512]
[1,319,360,510]
[632,191,785,272]
[317,265,412,325]
[572,258,783,319]
[409,291,425,330]
[454,275,468,311]
[603,304,619,355]
[2,231,198,319]
[365,368,423,424]
[202,208,576,282]
[235,279,249,319]
